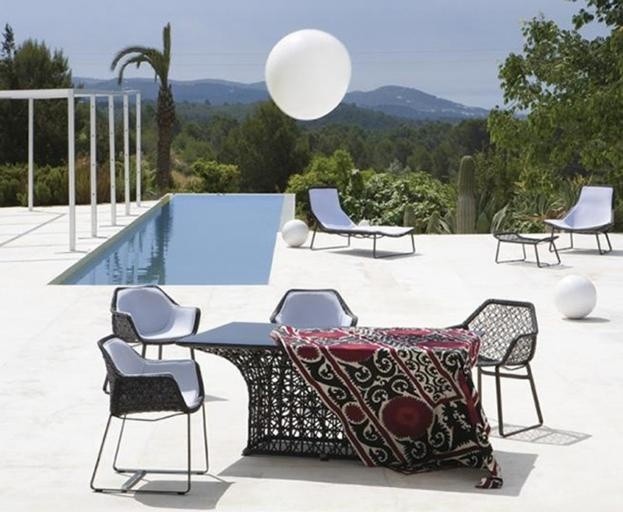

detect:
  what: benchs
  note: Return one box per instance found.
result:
[493,230,561,269]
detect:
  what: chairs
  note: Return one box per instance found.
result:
[542,183,614,255]
[102,286,199,396]
[89,336,208,495]
[446,299,542,437]
[307,188,416,259]
[271,288,359,329]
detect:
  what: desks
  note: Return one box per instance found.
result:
[175,322,484,464]
